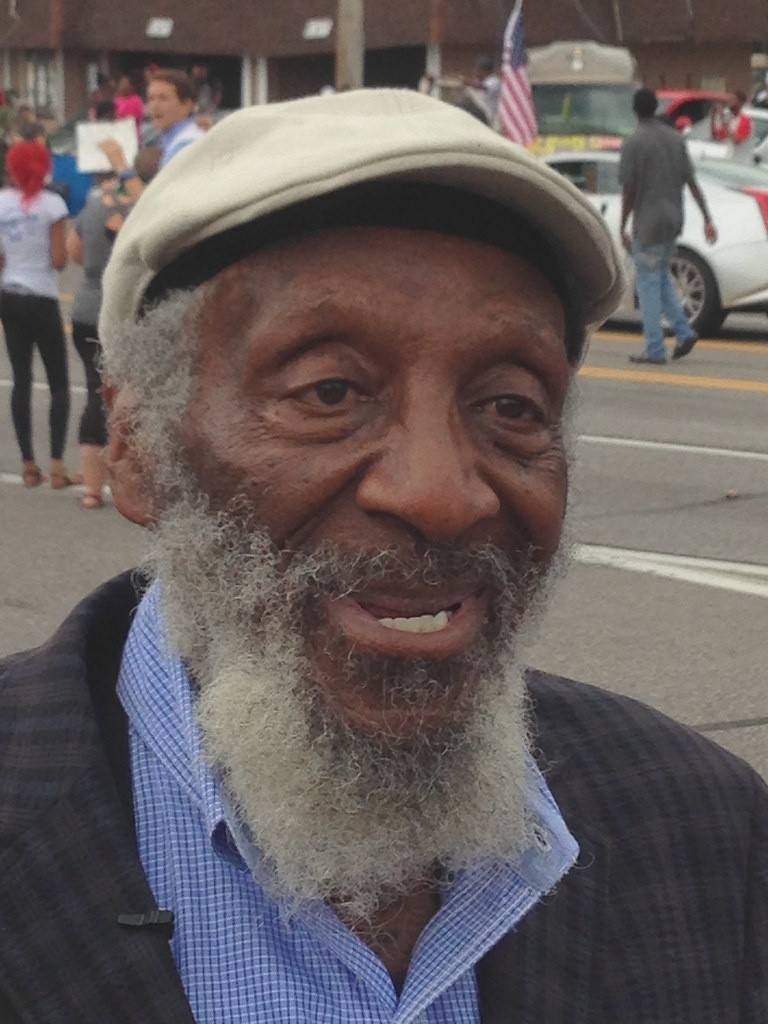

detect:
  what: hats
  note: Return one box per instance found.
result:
[98,88,628,370]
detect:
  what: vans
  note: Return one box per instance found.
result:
[519,74,644,164]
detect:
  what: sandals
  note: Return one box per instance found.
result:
[82,494,103,510]
[21,467,42,486]
[49,472,85,488]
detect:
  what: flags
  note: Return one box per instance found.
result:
[499,5,538,145]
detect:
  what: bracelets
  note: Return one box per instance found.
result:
[121,169,134,181]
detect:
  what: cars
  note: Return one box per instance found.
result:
[650,85,743,128]
[535,149,768,338]
[684,111,767,154]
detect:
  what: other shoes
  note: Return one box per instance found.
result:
[673,333,698,358]
[631,353,665,364]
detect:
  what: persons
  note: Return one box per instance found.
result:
[0,86,768,1024]
[674,115,692,135]
[619,90,718,362]
[0,60,208,509]
[710,89,753,144]
